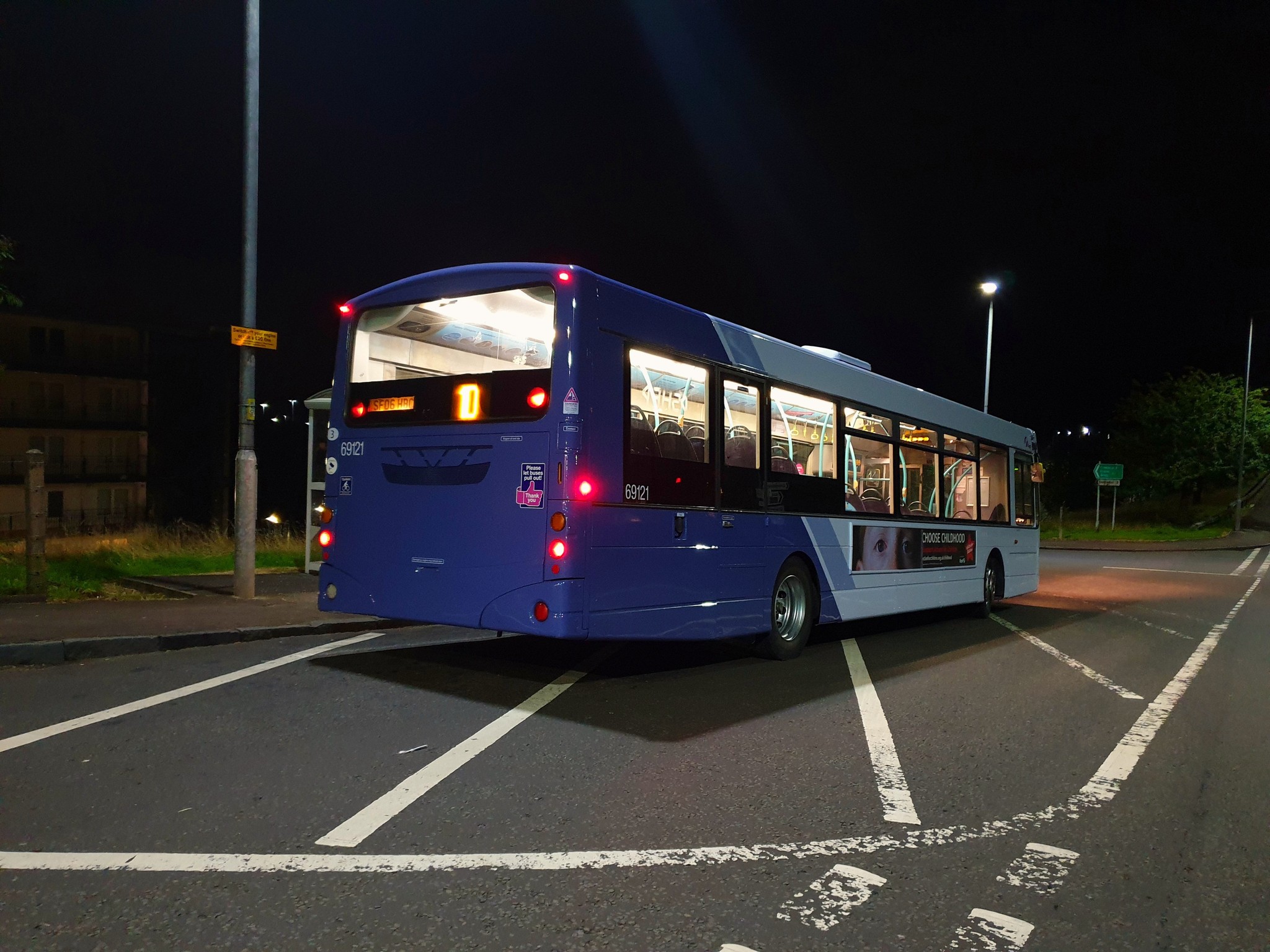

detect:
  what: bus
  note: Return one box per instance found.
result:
[318,262,1046,660]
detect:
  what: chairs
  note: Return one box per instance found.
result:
[846,476,974,519]
[628,404,802,476]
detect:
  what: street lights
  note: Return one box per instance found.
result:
[981,282,997,414]
